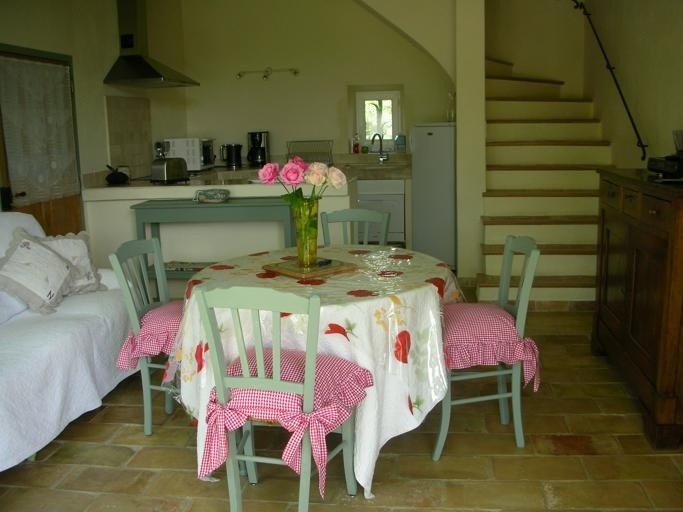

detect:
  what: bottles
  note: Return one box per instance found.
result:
[351,132,359,155]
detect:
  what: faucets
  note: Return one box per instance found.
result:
[371,133,384,158]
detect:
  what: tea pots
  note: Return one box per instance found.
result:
[218,142,243,170]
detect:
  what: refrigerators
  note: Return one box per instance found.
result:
[403,122,454,271]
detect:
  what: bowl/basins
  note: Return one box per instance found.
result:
[196,189,229,203]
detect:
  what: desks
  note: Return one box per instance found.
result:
[186,246,454,495]
[130,196,297,300]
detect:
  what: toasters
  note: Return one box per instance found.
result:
[149,158,190,184]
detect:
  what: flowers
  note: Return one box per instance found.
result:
[259,156,347,198]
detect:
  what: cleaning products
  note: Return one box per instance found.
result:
[354,133,360,152]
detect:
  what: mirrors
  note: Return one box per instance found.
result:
[355,90,401,155]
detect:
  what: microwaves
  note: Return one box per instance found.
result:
[162,137,216,172]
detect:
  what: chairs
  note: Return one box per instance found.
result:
[195,287,357,511]
[321,208,390,247]
[109,237,192,434]
[433,236,539,462]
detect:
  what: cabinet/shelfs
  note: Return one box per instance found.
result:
[594,169,683,448]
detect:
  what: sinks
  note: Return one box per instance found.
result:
[344,160,409,179]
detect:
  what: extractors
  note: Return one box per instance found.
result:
[101,0,200,90]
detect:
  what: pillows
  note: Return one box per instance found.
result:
[0,228,79,317]
[34,231,108,294]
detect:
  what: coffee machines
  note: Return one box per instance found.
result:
[245,131,269,164]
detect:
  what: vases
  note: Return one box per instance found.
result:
[290,198,319,266]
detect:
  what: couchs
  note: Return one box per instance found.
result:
[0,208,145,478]
